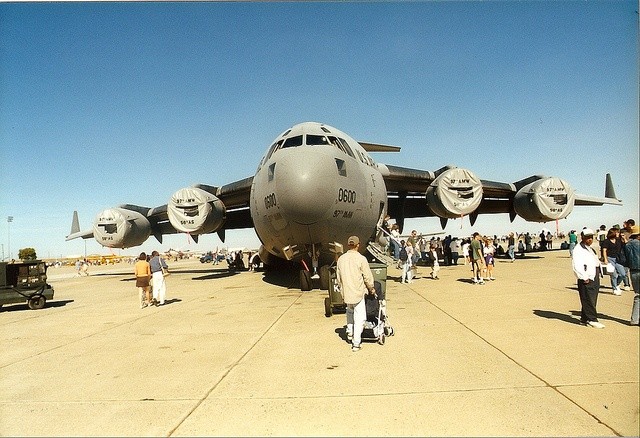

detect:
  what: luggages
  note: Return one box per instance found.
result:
[144,280,152,293]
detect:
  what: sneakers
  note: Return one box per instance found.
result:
[580,318,586,325]
[511,258,515,263]
[435,276,440,280]
[430,272,434,278]
[347,334,354,340]
[141,304,148,309]
[490,277,496,280]
[617,286,620,290]
[630,320,640,325]
[353,344,364,352]
[148,303,153,307]
[612,289,621,296]
[486,277,490,280]
[625,286,630,291]
[479,280,484,284]
[472,280,477,283]
[586,320,605,329]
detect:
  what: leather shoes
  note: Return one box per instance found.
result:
[153,298,157,306]
[159,302,165,306]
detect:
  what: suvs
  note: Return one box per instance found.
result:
[1,260,54,311]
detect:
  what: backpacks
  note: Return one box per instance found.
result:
[399,246,408,261]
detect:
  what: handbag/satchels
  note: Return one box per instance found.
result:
[158,256,170,277]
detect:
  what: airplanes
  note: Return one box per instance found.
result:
[64,122,625,291]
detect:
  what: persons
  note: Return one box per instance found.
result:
[81,259,89,276]
[407,230,420,267]
[230,250,260,272]
[91,257,135,265]
[620,218,635,233]
[596,225,607,261]
[568,230,576,257]
[400,240,412,284]
[397,241,413,269]
[620,225,640,326]
[429,244,440,280]
[383,215,391,255]
[135,252,152,308]
[429,236,435,249]
[173,250,224,265]
[425,240,431,257]
[443,232,504,285]
[539,230,547,250]
[48,260,75,268]
[562,241,567,249]
[525,232,532,251]
[391,224,399,261]
[420,241,427,262]
[336,236,375,351]
[602,228,625,295]
[508,232,515,262]
[149,251,168,307]
[546,232,553,249]
[75,260,81,276]
[436,237,442,255]
[618,230,631,290]
[571,228,605,329]
[518,240,525,256]
[623,222,627,226]
[533,243,539,250]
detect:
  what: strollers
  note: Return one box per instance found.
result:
[345,282,394,345]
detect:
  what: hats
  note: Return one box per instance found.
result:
[630,225,640,235]
[348,236,361,245]
[600,225,606,228]
[581,229,596,237]
